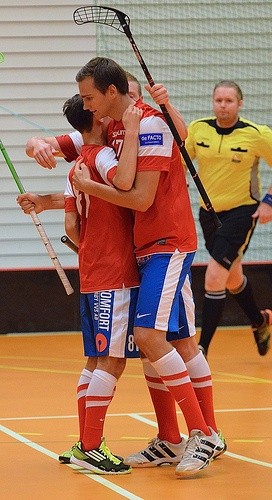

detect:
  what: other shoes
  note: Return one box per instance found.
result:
[169,429,227,464]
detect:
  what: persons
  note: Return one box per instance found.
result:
[15,58,226,477]
[181,81,272,362]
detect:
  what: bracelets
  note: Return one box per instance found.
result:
[262,194,272,206]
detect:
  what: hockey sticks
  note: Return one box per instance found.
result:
[73,5,223,230]
[0,51,74,296]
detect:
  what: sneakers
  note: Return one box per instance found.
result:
[59,442,79,463]
[252,309,272,355]
[198,345,207,360]
[175,426,224,472]
[70,436,132,474]
[122,434,186,468]
